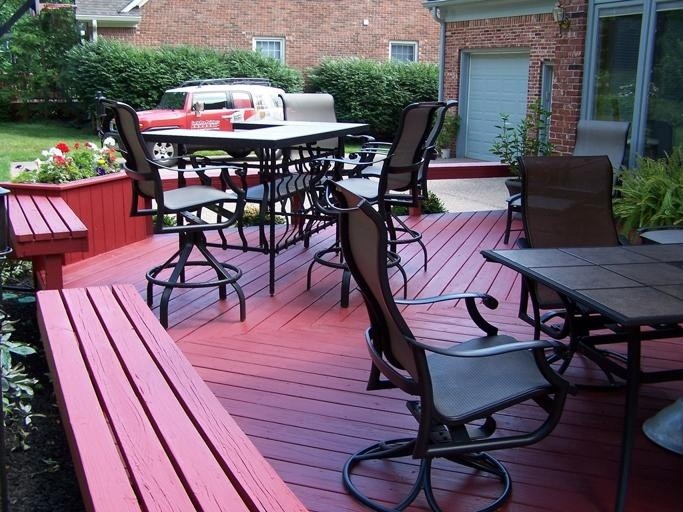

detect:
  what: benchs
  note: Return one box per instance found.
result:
[2,194,91,287]
[31,282,310,510]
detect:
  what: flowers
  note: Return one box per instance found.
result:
[9,134,120,185]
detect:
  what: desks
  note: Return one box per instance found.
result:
[149,118,370,297]
[478,239,683,512]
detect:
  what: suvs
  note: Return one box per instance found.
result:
[109,77,287,167]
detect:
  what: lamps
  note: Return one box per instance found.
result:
[552,0,572,35]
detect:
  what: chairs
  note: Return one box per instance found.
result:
[278,90,376,251]
[323,178,580,511]
[643,118,675,164]
[350,99,459,274]
[114,101,252,329]
[303,101,442,308]
[516,153,632,396]
